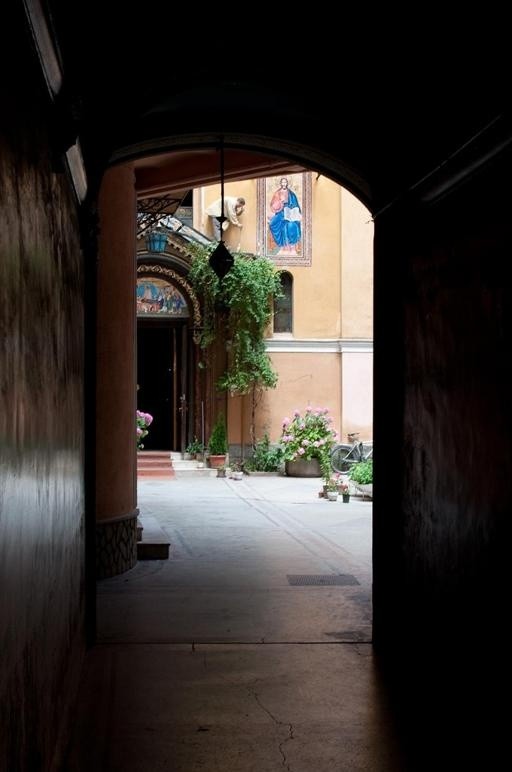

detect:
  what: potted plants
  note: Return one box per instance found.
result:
[318,456,372,505]
[205,411,230,470]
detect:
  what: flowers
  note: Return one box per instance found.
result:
[274,404,338,463]
[134,407,154,454]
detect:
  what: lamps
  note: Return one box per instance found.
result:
[144,220,171,255]
[205,124,236,289]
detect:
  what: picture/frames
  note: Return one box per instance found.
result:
[251,170,315,268]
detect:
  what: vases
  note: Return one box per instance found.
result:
[281,452,327,479]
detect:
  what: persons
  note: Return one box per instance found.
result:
[202,195,246,242]
[268,177,301,255]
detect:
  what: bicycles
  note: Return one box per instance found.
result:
[331,431,373,474]
